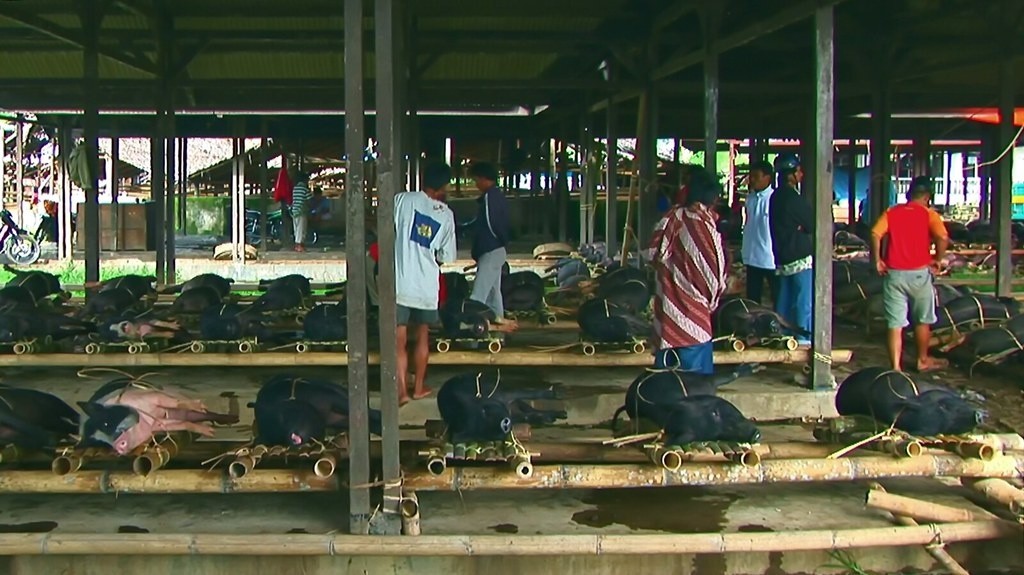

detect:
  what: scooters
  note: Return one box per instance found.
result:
[31,201,78,248]
[245,209,319,247]
[0,209,42,266]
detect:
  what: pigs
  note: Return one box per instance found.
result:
[576,298,652,342]
[252,275,315,312]
[833,221,1024,247]
[160,271,235,314]
[835,366,983,436]
[544,241,651,312]
[710,295,811,346]
[0,264,98,343]
[247,376,383,448]
[77,271,157,317]
[501,271,545,312]
[303,305,347,341]
[610,362,767,446]
[437,372,568,441]
[73,377,240,454]
[1,384,81,452]
[199,303,263,340]
[832,260,1024,370]
[440,272,495,340]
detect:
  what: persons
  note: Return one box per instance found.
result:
[859,176,948,372]
[292,173,335,251]
[394,162,457,405]
[471,165,510,318]
[650,174,728,374]
[742,153,813,343]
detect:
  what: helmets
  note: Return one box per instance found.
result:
[774,151,800,172]
